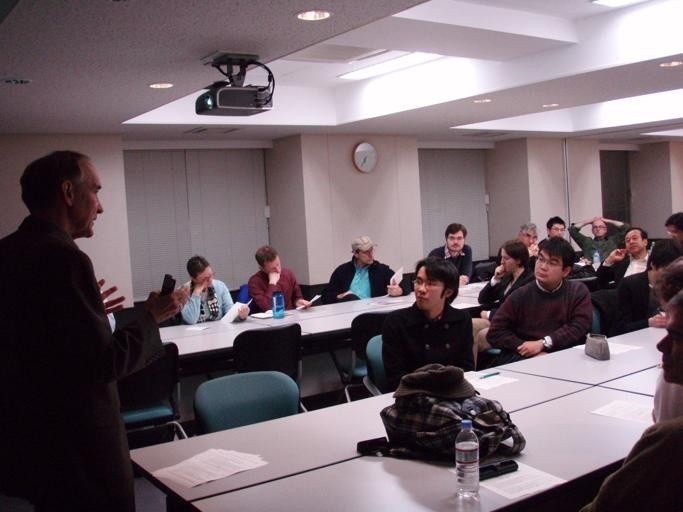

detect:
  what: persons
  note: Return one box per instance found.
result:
[321,235,411,304]
[382,256,476,391]
[485,236,593,369]
[248,246,312,312]
[652,256,683,423]
[471,240,535,370]
[497,213,683,314]
[177,256,250,325]
[426,223,472,285]
[578,294,683,512]
[0,150,189,512]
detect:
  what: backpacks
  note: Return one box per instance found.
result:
[357,396,526,462]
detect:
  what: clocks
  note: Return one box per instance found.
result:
[353,142,378,175]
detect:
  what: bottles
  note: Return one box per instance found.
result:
[455,420,479,498]
[272,291,284,319]
[650,242,655,253]
[594,250,600,268]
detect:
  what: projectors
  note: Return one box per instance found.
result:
[194,51,275,117]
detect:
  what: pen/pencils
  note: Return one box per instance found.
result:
[481,372,499,378]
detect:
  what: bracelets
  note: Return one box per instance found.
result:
[542,337,551,352]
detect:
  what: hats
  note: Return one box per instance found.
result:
[393,363,476,398]
[352,236,378,252]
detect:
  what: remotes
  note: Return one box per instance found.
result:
[458,460,518,483]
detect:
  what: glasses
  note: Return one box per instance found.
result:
[412,279,438,289]
